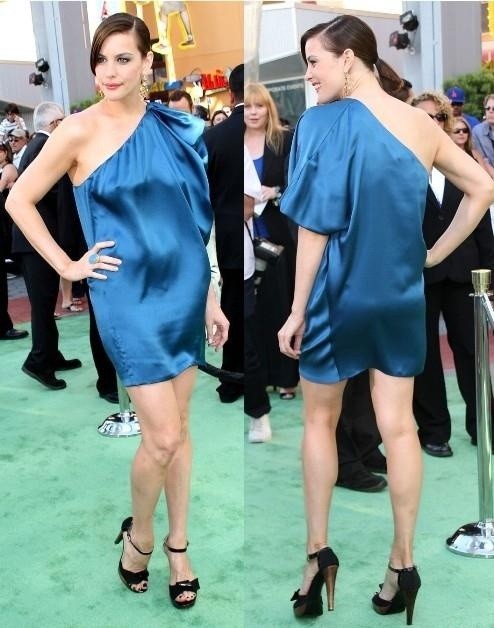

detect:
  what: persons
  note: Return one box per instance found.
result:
[277,12,494,620]
[3,12,233,609]
[0,65,493,496]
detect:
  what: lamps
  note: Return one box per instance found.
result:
[27,57,49,88]
[388,10,420,54]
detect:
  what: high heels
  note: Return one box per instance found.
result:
[371,563,422,626]
[291,547,339,619]
[163,534,200,610]
[114,517,154,593]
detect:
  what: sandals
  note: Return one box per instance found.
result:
[272,384,296,400]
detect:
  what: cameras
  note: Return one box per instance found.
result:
[252,238,284,299]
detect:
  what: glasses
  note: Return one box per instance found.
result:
[484,107,494,111]
[452,128,468,134]
[429,113,447,121]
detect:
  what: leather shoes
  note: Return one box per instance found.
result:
[22,353,67,389]
[54,351,81,371]
[1,327,29,339]
[425,441,453,457]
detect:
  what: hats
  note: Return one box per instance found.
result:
[7,129,26,138]
[445,87,465,103]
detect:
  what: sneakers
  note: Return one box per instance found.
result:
[369,450,387,474]
[335,472,387,493]
[247,413,271,444]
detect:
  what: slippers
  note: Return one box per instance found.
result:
[72,297,82,304]
[62,304,83,312]
